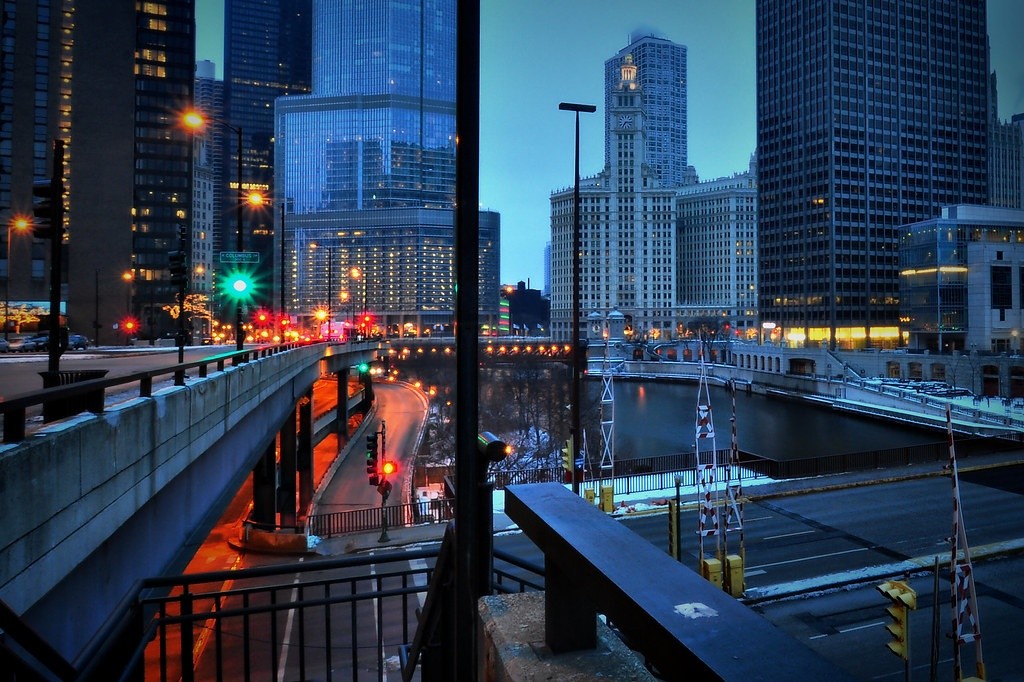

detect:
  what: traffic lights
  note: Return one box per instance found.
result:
[561,440,572,472]
[366,434,397,474]
[876,580,917,662]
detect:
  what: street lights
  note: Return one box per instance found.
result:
[95,266,132,348]
[340,292,355,326]
[358,364,370,411]
[4,220,29,341]
[351,269,367,340]
[245,192,287,346]
[507,287,526,337]
[180,110,246,352]
[309,241,331,341]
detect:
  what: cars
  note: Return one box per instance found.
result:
[0,331,90,353]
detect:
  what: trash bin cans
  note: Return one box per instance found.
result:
[38,369,110,423]
